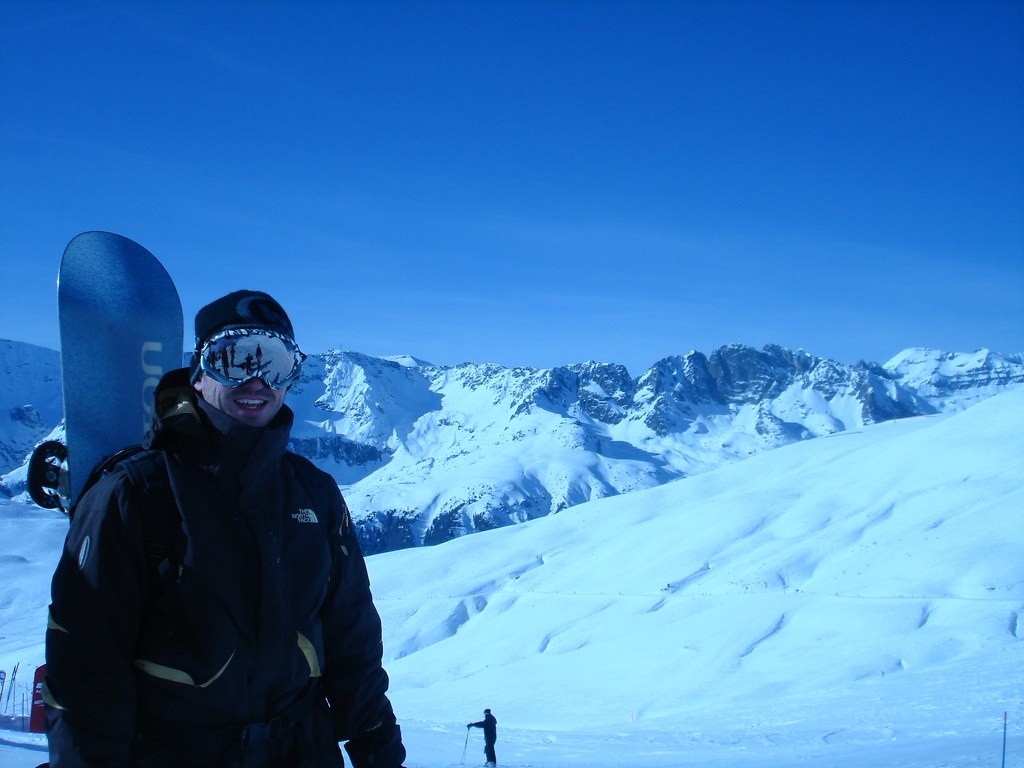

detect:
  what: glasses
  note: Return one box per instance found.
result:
[194,328,306,389]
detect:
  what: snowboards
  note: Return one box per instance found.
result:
[55,229,183,522]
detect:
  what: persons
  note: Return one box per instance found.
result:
[44,289,407,768]
[466,709,497,768]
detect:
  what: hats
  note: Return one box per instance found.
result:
[193,289,295,362]
